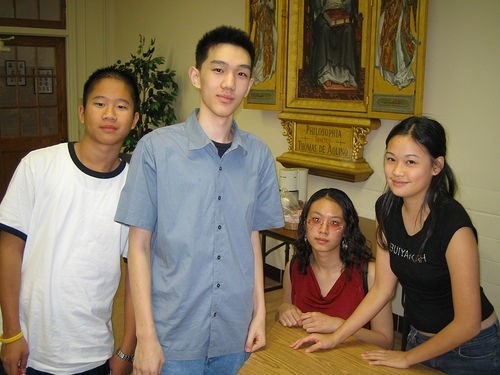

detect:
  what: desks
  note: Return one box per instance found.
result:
[235,316,445,375]
[259,214,377,294]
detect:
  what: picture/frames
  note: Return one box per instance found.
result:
[33,68,53,94]
[6,60,26,86]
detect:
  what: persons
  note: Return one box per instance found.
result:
[277,189,395,351]
[0,65,139,375]
[115,26,287,375]
[290,117,500,374]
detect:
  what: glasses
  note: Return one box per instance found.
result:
[303,218,345,231]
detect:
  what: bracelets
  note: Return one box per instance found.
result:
[116,349,134,361]
[0,331,24,344]
